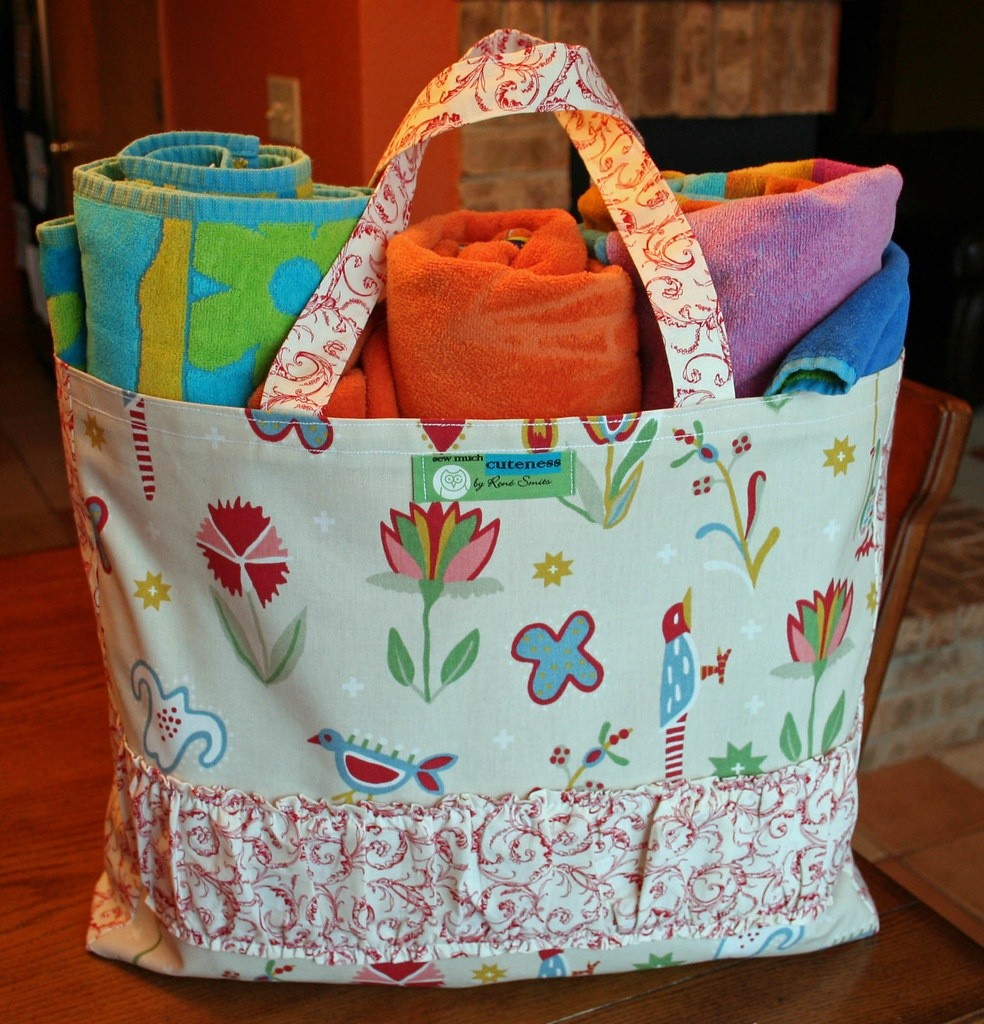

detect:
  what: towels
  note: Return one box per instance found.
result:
[34,128,910,423]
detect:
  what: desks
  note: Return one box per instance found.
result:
[0,543,984,1024]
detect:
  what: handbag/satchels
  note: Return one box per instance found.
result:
[57,27,905,982]
